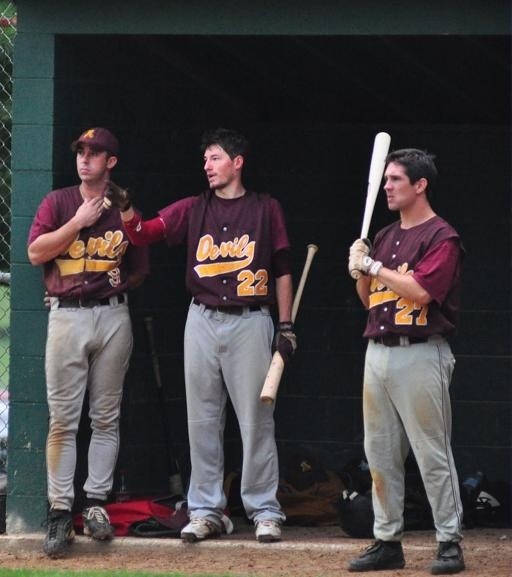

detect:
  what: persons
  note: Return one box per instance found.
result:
[346,148,467,576]
[25,127,149,560]
[100,126,299,544]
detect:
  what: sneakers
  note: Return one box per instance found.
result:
[41,510,75,557]
[430,536,465,574]
[253,519,282,542]
[181,518,221,541]
[350,539,405,571]
[81,500,115,541]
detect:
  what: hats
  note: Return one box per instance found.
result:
[71,128,117,155]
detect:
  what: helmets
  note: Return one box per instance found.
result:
[474,491,501,525]
[340,489,373,537]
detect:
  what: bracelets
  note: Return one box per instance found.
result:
[369,261,381,278]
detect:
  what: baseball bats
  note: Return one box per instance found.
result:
[351,131,393,280]
[143,316,184,497]
[260,244,316,405]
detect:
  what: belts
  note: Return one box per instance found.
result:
[369,334,428,346]
[59,294,124,307]
[193,298,260,315]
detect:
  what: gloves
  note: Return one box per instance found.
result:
[102,180,131,211]
[272,321,297,364]
[348,238,383,278]
